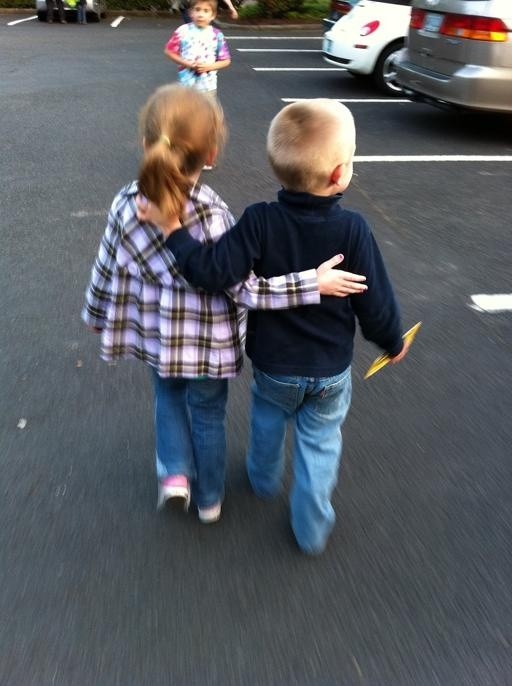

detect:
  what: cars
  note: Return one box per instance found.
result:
[321,0,512,124]
[36,0,107,22]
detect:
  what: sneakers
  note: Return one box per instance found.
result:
[157,475,221,523]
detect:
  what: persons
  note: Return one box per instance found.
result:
[135,98,405,559]
[164,0,231,167]
[223,0,239,23]
[81,85,370,525]
